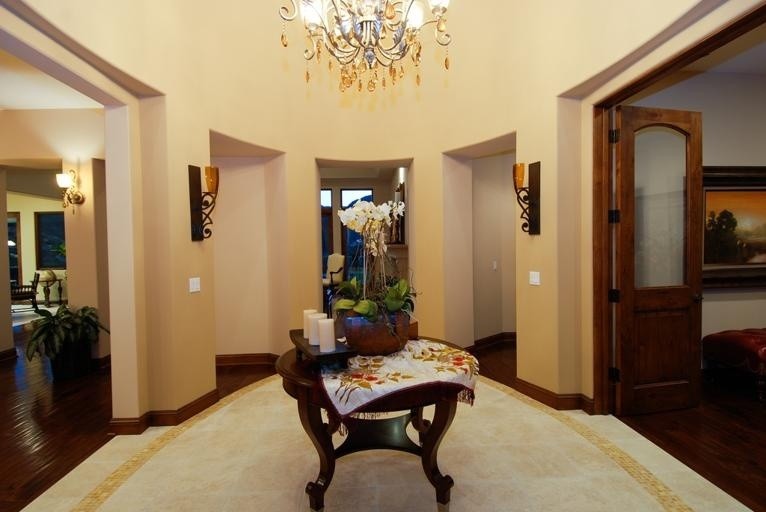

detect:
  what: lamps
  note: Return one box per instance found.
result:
[189,164,220,242]
[55,168,84,212]
[512,161,541,237]
[278,1,452,95]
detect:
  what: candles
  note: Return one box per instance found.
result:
[302,307,336,352]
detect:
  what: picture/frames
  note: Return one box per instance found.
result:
[703,166,765,291]
[393,183,405,240]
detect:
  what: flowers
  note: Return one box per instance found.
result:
[333,199,419,322]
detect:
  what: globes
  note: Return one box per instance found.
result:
[30,269,63,308]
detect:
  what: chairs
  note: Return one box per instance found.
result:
[11,273,39,308]
[322,254,345,289]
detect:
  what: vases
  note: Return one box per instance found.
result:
[340,310,409,354]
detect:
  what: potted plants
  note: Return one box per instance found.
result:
[25,305,111,386]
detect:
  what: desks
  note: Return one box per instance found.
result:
[274,331,479,512]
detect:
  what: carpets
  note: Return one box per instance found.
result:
[11,305,67,328]
[15,373,752,511]
